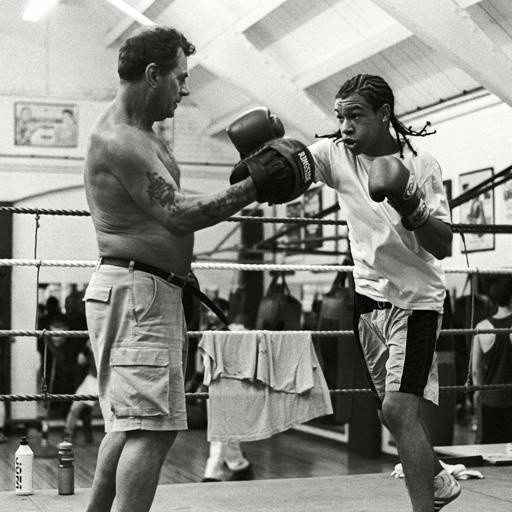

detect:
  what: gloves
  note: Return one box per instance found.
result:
[226,106,315,206]
[368,156,431,231]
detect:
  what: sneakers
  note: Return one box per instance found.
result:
[433,469,461,512]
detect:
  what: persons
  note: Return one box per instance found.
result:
[82,26,318,511]
[224,73,461,512]
[37,283,512,482]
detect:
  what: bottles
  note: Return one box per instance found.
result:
[15,437,33,497]
[57,438,74,495]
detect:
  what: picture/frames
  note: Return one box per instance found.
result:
[457,167,497,254]
[285,186,324,256]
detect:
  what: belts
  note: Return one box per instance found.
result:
[363,300,393,310]
[99,256,229,326]
